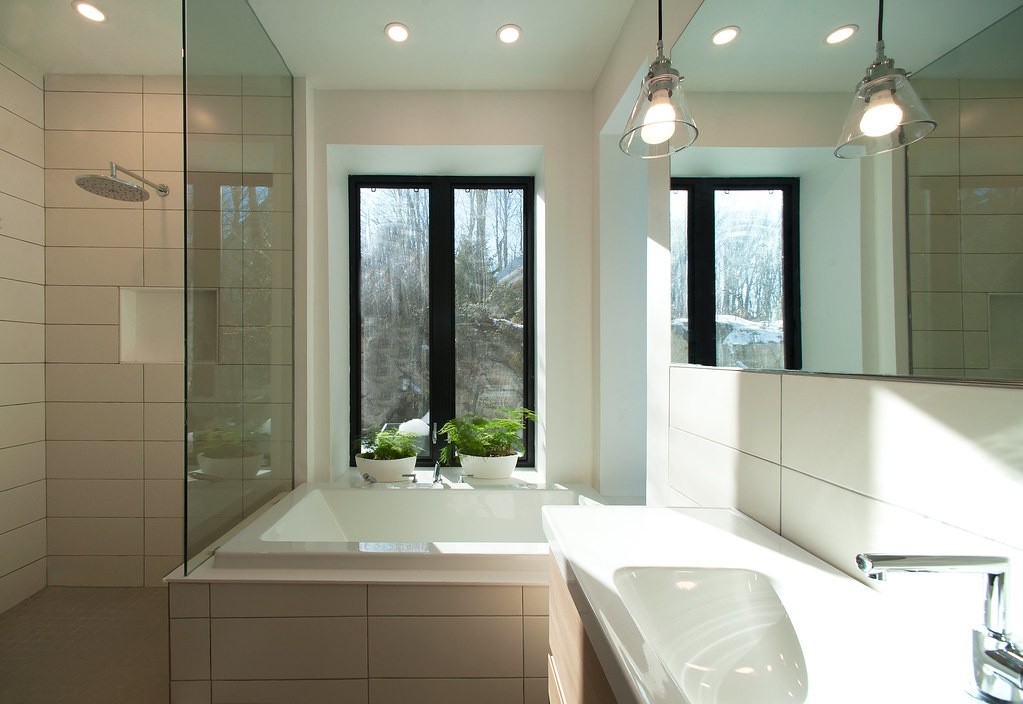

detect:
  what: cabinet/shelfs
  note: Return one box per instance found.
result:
[548,547,618,704]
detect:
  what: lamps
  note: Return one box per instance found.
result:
[618,0,700,159]
[833,0,937,158]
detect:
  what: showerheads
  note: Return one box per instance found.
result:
[75,174,150,202]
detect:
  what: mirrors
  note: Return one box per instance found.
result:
[668,0,1023,390]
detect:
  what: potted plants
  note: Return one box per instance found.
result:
[351,426,424,481]
[440,407,539,479]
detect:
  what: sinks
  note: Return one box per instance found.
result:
[611,562,808,704]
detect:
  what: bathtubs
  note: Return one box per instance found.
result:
[162,483,612,704]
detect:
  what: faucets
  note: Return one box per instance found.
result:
[433,461,443,484]
[855,553,1023,704]
